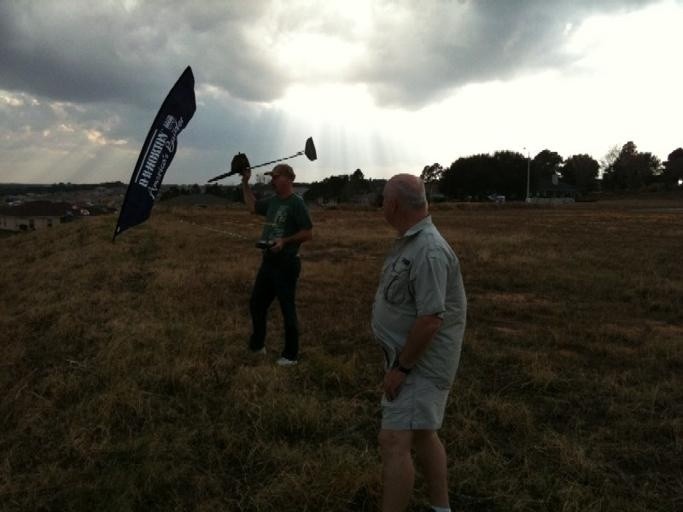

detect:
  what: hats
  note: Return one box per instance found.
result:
[264,165,295,181]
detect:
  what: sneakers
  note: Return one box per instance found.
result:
[275,357,298,367]
[248,345,267,358]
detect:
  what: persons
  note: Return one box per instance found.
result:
[238,165,313,367]
[371,174,469,512]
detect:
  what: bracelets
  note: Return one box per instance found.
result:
[398,365,412,375]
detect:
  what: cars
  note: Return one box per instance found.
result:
[495,196,506,203]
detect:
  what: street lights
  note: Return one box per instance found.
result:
[523,147,530,197]
